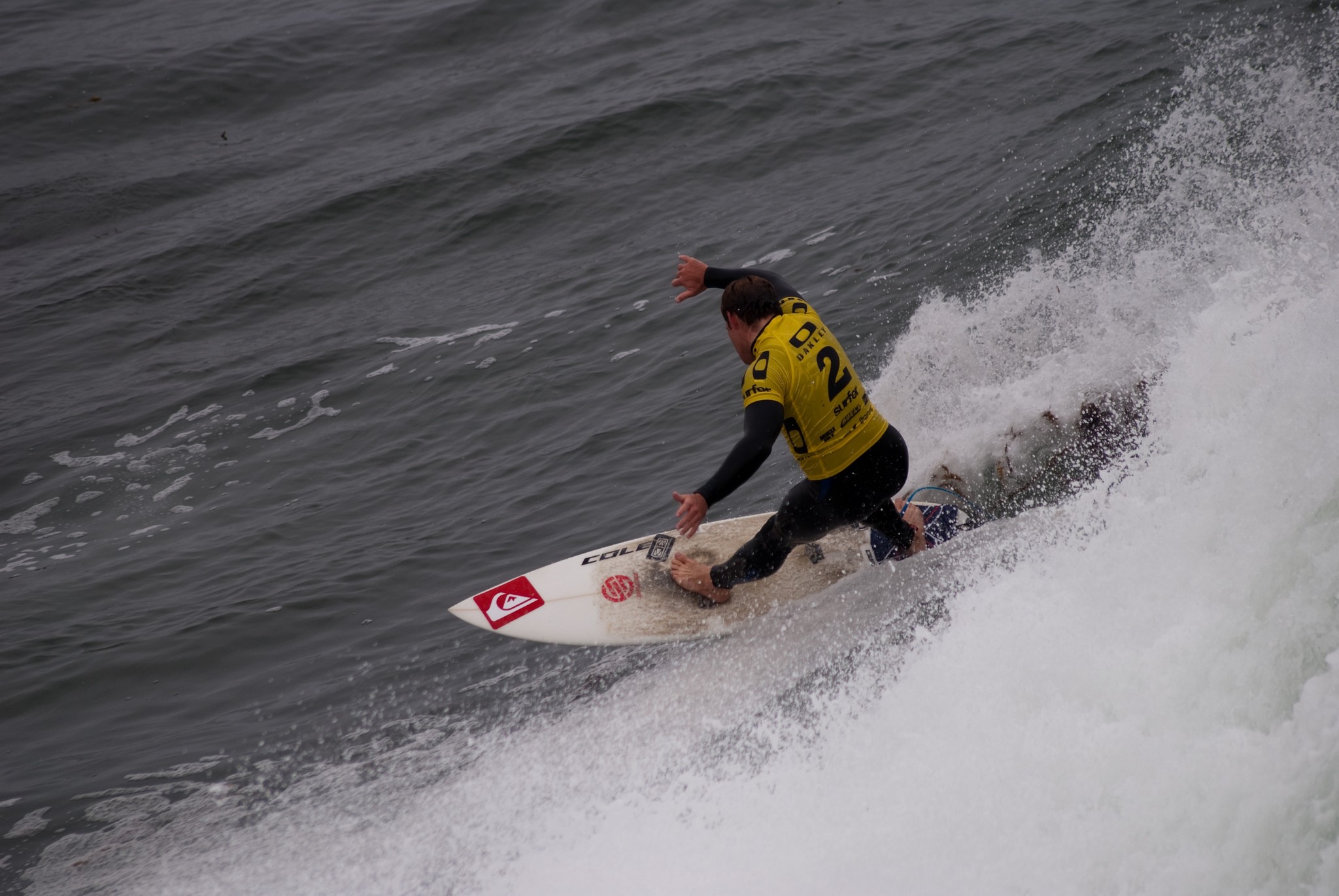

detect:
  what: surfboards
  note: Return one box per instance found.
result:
[447,501,976,647]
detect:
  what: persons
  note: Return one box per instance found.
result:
[668,256,924,603]
